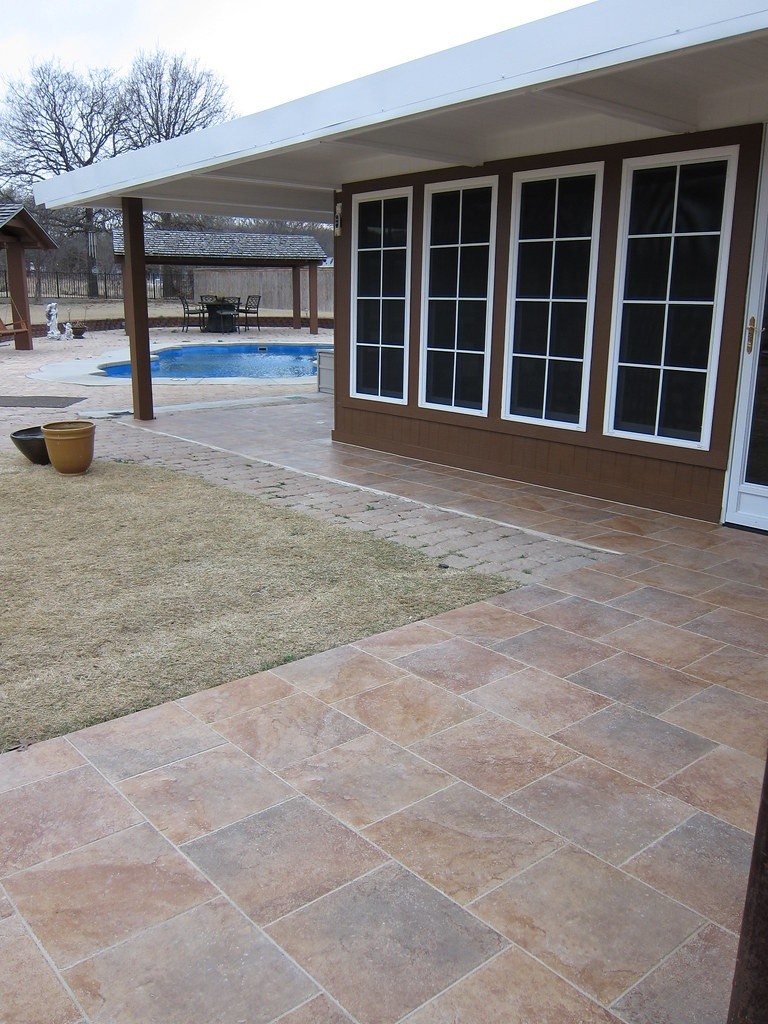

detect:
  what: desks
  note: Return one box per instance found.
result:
[198,301,242,333]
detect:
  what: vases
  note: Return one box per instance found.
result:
[10,427,50,466]
[41,421,96,476]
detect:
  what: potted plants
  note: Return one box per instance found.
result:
[71,320,87,339]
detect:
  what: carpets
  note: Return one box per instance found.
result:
[0,395,88,409]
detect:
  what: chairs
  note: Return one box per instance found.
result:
[178,293,262,335]
